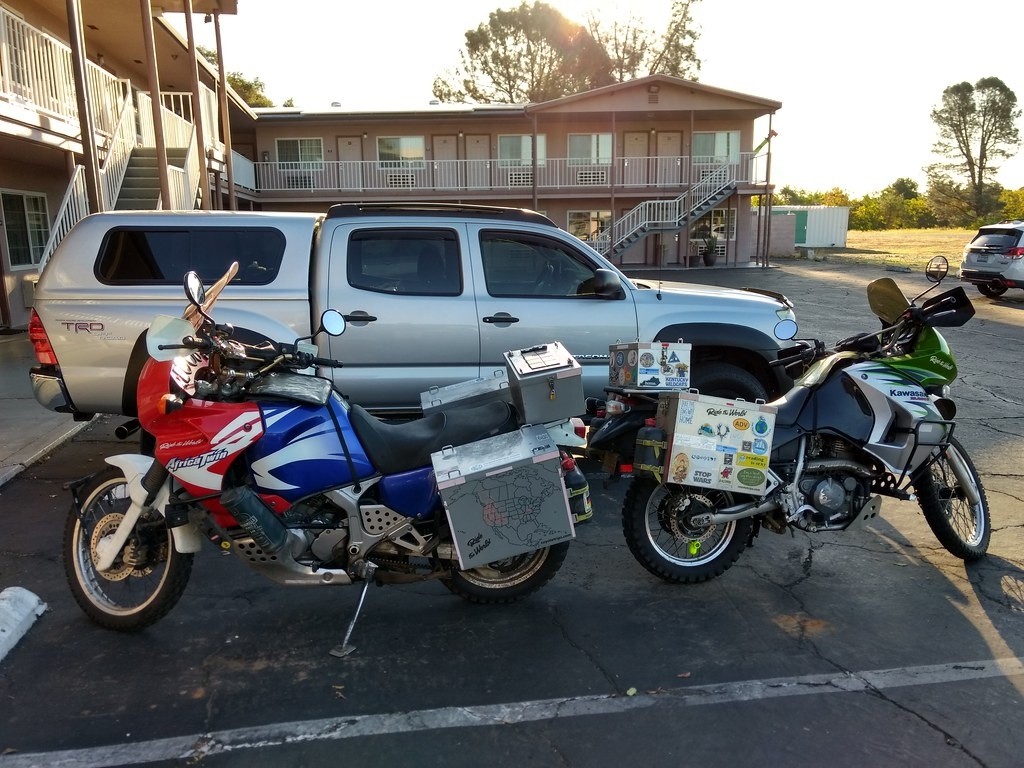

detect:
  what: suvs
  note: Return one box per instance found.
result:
[958,216,1024,298]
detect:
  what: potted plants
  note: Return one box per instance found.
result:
[702,233,718,267]
[683,241,700,267]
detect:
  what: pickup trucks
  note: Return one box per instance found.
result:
[29,201,802,464]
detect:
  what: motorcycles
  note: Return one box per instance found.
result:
[61,257,596,658]
[581,254,993,584]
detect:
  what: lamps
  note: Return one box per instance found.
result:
[651,128,656,133]
[97,53,104,64]
[204,13,212,24]
[363,132,368,138]
[648,85,660,93]
[459,130,463,137]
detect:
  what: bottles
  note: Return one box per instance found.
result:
[583,412,607,459]
[561,450,594,523]
[630,417,667,479]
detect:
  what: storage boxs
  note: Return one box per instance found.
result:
[609,340,691,389]
[656,387,776,495]
[421,372,508,413]
[431,425,576,569]
[504,341,587,424]
